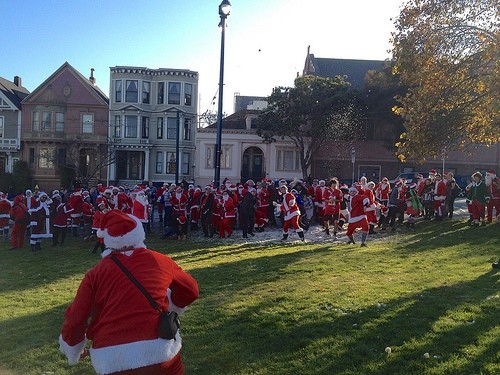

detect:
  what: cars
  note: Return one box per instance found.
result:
[389,172,428,189]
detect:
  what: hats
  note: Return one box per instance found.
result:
[1,173,151,204]
[486,169,496,176]
[162,173,448,200]
[97,207,145,250]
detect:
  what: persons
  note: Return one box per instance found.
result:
[0,169,500,271]
[60,208,200,375]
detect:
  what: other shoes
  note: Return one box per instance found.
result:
[465,217,500,228]
[0,228,10,239]
[7,245,24,251]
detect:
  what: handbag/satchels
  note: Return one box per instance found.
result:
[159,308,181,340]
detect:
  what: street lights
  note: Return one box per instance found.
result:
[213,0,232,189]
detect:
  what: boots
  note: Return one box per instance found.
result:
[29,240,42,252]
[151,212,378,247]
[92,243,106,254]
[381,210,454,230]
[51,219,98,249]
